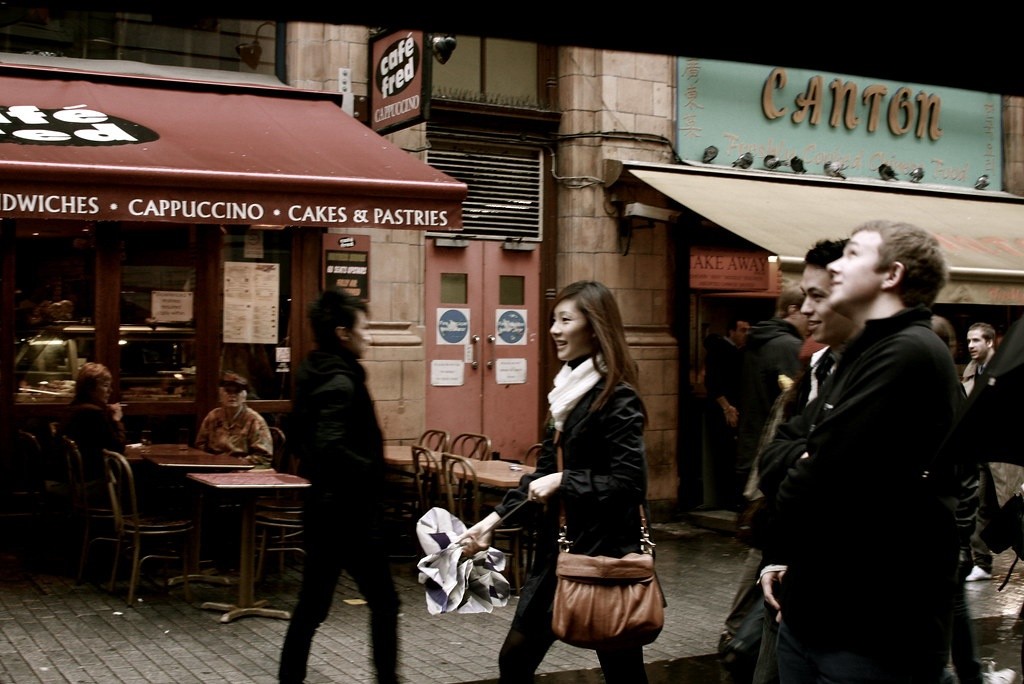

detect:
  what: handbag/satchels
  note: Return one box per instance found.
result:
[550,551,667,647]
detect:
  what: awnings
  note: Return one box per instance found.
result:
[602,158,1024,308]
[0,52,468,232]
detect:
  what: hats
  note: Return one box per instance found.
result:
[218,372,249,387]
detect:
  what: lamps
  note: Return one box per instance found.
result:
[431,32,458,63]
[235,20,277,69]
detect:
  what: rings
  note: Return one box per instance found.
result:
[532,497,538,499]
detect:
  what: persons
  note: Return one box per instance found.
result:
[70,362,126,507]
[195,373,273,470]
[277,289,401,684]
[706,221,1024,684]
[459,281,668,684]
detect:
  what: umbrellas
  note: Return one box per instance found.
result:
[416,497,530,615]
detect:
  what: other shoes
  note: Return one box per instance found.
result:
[965,565,993,581]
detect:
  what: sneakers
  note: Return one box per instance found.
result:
[982,660,1016,684]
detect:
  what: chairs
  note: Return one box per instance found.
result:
[103,448,195,606]
[255,427,542,595]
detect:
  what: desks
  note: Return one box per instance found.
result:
[186,471,311,622]
[420,459,536,574]
[383,445,479,514]
[141,447,255,585]
